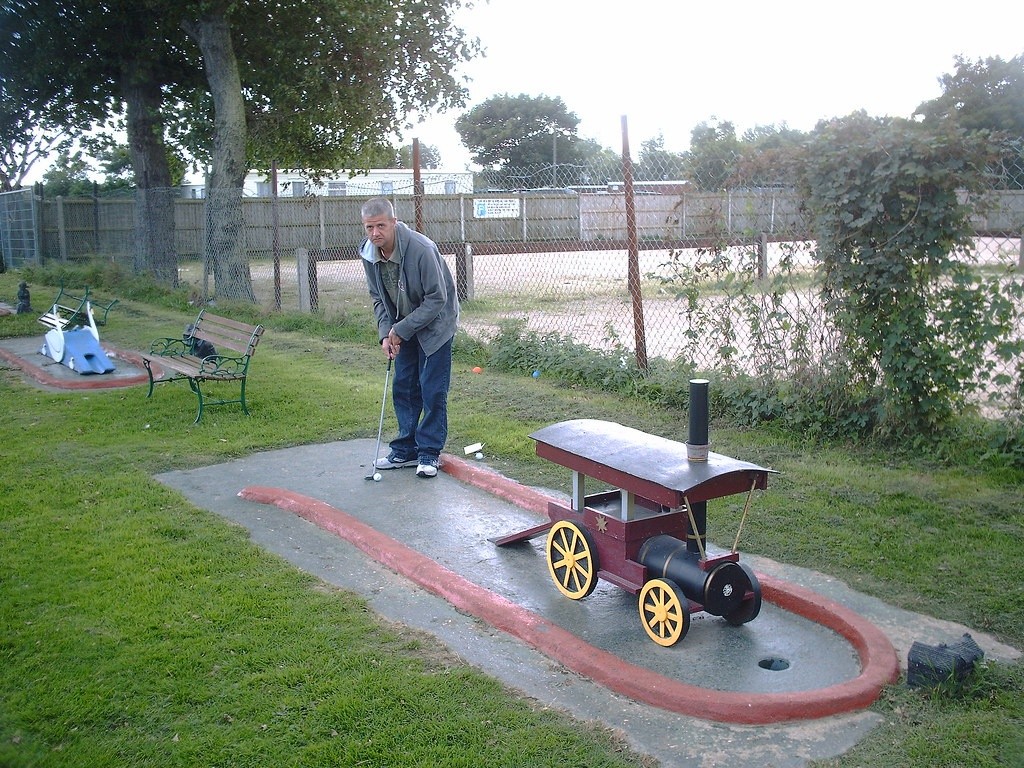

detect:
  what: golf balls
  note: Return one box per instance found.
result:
[476,453,483,459]
[374,473,382,482]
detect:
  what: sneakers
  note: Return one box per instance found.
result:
[373,452,421,469]
[415,458,440,478]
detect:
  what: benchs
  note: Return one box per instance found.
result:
[141,310,265,424]
[37,280,120,332]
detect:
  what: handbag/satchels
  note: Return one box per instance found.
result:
[188,337,217,360]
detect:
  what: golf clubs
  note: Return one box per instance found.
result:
[363,343,393,480]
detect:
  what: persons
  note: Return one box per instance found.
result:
[17,282,30,314]
[182,324,216,362]
[359,197,459,478]
